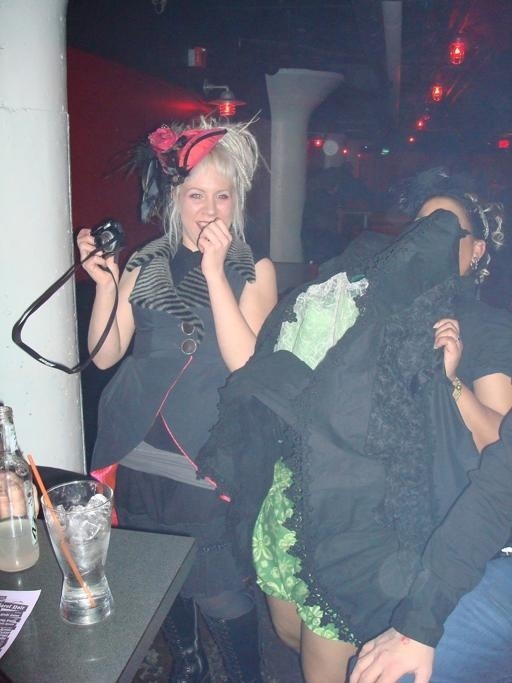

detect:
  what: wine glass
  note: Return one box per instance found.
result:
[40,479,117,625]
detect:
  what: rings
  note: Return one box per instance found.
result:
[456,333,463,342]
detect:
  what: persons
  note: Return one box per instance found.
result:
[75,110,282,681]
[345,404,512,682]
[249,183,511,681]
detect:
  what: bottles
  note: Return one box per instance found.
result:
[0,405,40,574]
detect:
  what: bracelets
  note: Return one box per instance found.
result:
[449,375,463,402]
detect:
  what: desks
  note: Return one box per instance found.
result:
[0,518,200,683]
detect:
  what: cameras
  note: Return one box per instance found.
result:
[90,219,126,258]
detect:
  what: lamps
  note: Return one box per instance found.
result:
[310,134,325,147]
[407,34,470,144]
[202,78,247,116]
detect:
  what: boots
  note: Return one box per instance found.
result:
[162,597,211,683]
[197,603,263,682]
